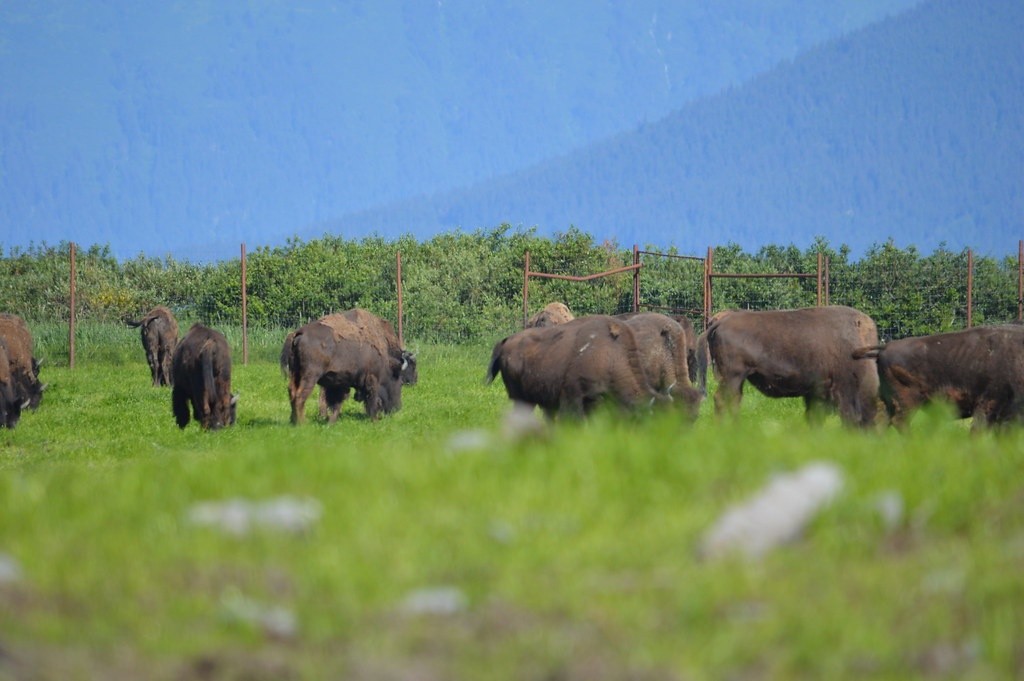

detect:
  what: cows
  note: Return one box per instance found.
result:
[482,301,1024,439]
[170,323,237,431]
[121,304,180,388]
[0,314,47,430]
[280,308,420,427]
